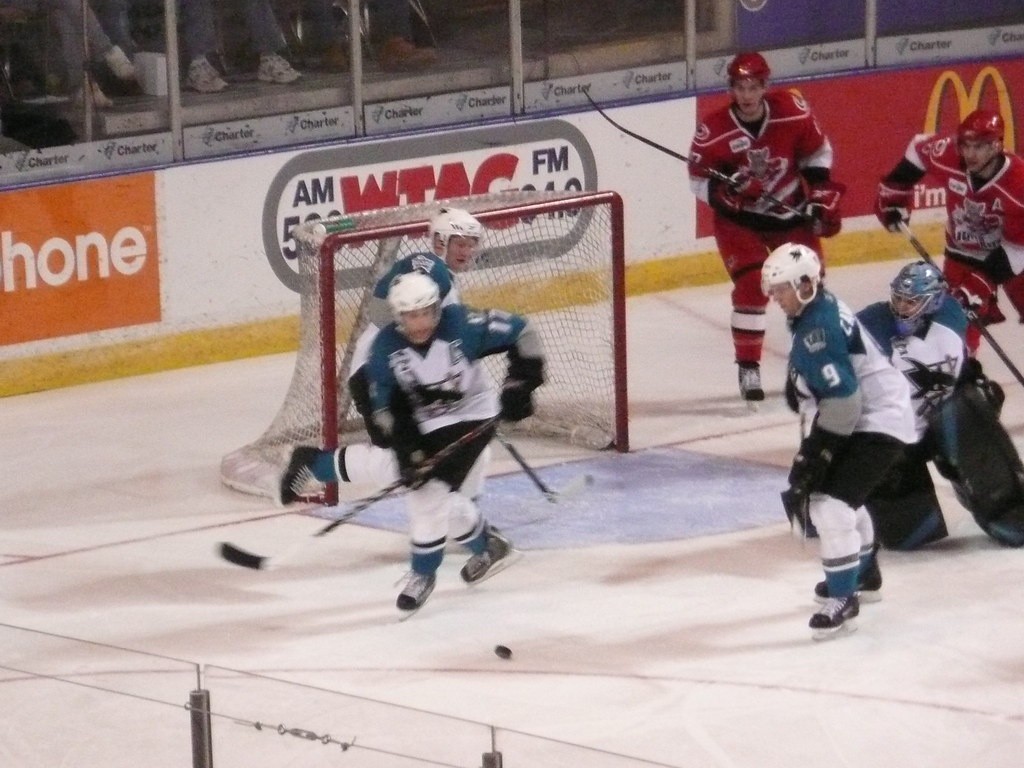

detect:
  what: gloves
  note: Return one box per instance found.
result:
[952,269,992,322]
[873,175,914,233]
[501,376,534,422]
[787,438,834,489]
[395,442,433,490]
[807,182,846,237]
[785,377,799,412]
[729,171,764,202]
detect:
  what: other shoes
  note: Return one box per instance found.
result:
[103,46,139,81]
[77,85,112,109]
[382,41,441,69]
[321,45,347,74]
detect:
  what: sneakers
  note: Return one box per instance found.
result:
[814,543,882,603]
[737,361,764,414]
[184,61,230,93]
[809,590,860,639]
[461,527,523,589]
[257,55,301,84]
[396,565,435,624]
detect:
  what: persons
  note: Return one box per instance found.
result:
[0,0,422,110]
[688,52,846,412]
[793,261,1024,553]
[874,110,1024,358]
[276,202,491,505]
[760,242,915,641]
[364,270,545,621]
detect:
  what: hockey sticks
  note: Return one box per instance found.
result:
[219,412,504,572]
[495,426,594,505]
[581,86,814,225]
[781,447,833,550]
[897,220,1024,388]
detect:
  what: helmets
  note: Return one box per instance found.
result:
[958,110,1004,144]
[761,242,820,290]
[889,260,948,337]
[386,272,440,313]
[729,52,770,81]
[430,207,483,243]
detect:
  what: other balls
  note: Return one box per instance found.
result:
[494,645,512,660]
